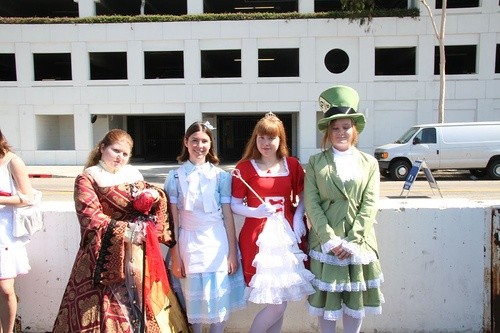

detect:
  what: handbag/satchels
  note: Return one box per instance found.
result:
[6,155,43,238]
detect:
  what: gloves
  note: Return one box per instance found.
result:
[229,196,274,218]
[292,192,307,245]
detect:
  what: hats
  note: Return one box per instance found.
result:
[318,85,366,134]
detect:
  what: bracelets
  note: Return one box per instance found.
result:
[130,228,137,245]
[147,187,160,204]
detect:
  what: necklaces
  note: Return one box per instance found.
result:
[261,157,279,173]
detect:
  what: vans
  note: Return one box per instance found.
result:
[373,120,500,181]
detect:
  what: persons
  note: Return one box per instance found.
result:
[0,130,34,333]
[162,121,238,333]
[230,116,309,333]
[303,111,380,333]
[73,128,180,333]
